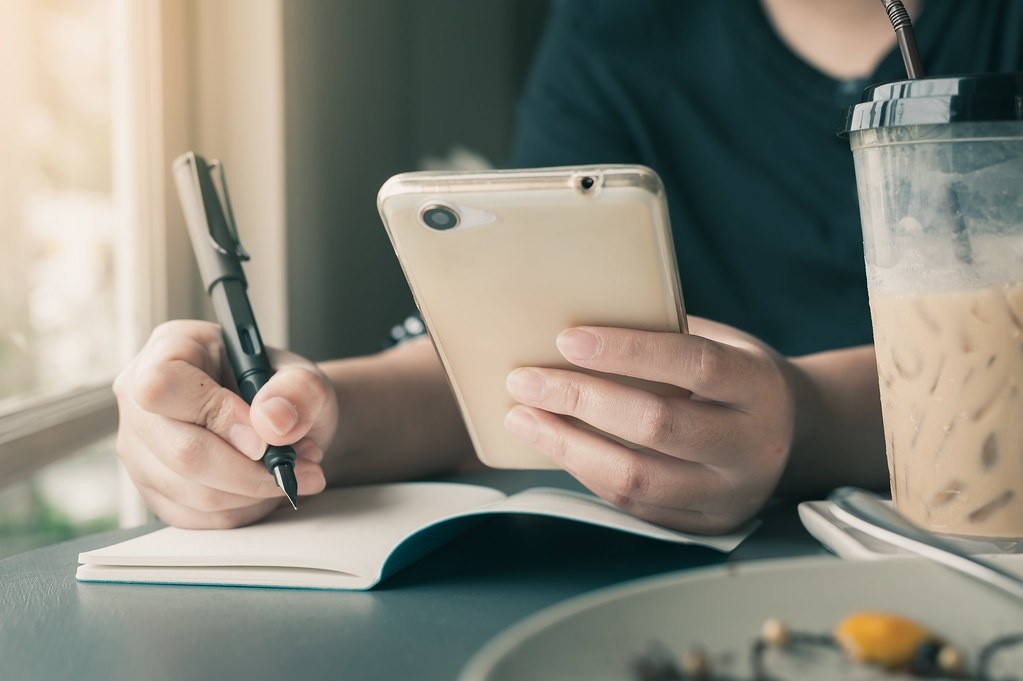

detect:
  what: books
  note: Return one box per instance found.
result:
[74,482,763,592]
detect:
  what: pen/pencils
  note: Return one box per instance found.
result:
[168,150,299,512]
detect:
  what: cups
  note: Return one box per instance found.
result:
[837,74,1023,553]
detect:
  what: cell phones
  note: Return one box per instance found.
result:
[378,165,690,471]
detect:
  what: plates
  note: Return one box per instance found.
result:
[457,550,1023,681]
[796,500,1002,559]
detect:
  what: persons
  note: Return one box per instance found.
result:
[109,0,1023,538]
